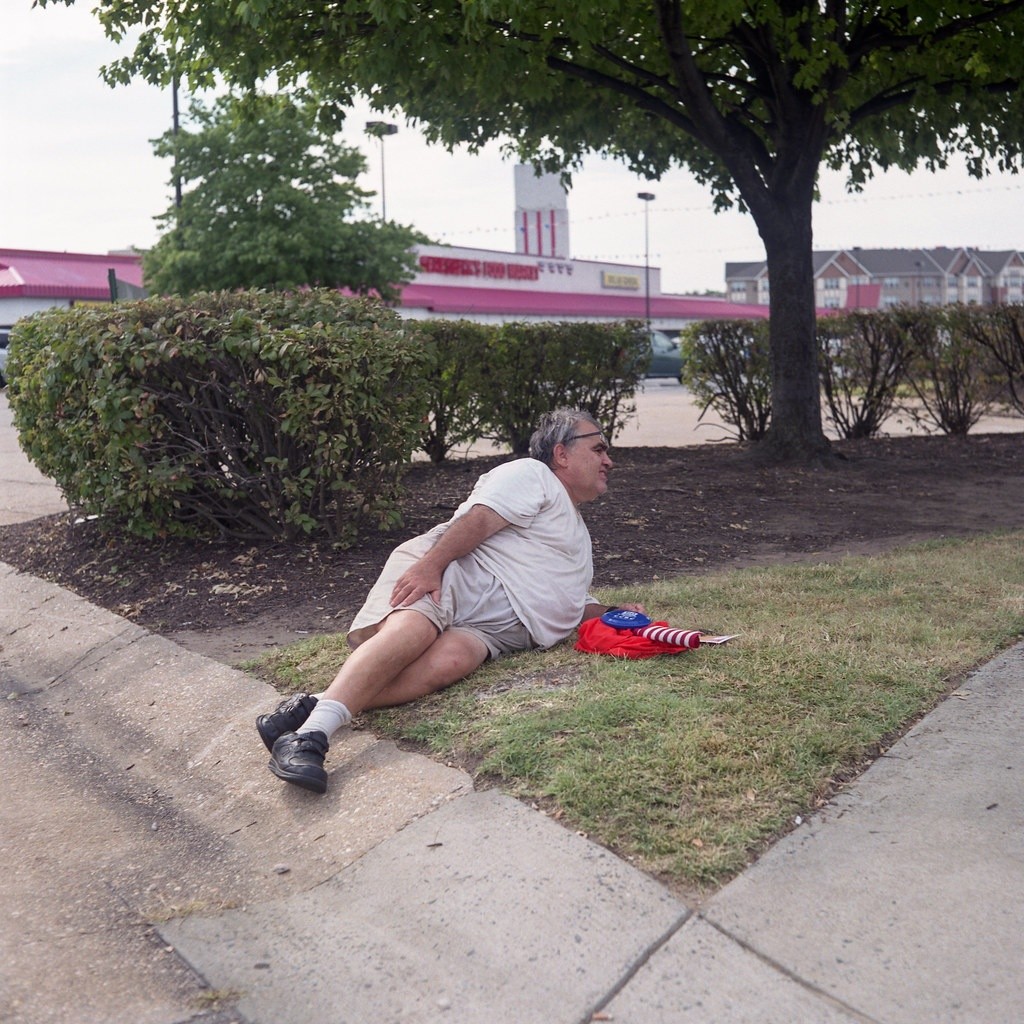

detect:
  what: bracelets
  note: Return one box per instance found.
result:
[605,606,619,613]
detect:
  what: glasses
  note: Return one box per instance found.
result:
[564,432,609,448]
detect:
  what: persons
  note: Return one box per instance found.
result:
[255,404,612,795]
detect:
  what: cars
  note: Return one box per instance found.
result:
[628,329,687,386]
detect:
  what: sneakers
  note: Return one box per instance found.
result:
[268,730,330,793]
[256,693,319,752]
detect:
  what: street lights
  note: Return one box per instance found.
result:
[365,122,397,222]
[852,246,863,313]
[915,260,922,306]
[637,193,656,330]
